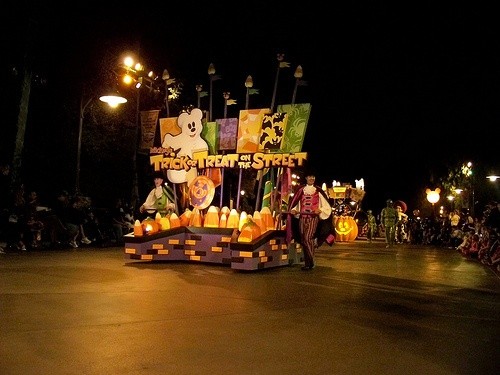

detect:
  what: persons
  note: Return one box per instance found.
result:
[287,171,332,271]
[139,173,176,219]
[333,198,500,273]
[0,186,148,255]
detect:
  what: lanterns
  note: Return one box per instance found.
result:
[333,216,358,242]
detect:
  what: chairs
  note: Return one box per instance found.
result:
[47,220,59,240]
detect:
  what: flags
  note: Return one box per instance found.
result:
[140,111,159,148]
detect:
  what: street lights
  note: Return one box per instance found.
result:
[76,90,128,188]
[113,57,158,137]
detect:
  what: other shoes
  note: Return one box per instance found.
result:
[0,233,126,256]
[302,263,314,270]
